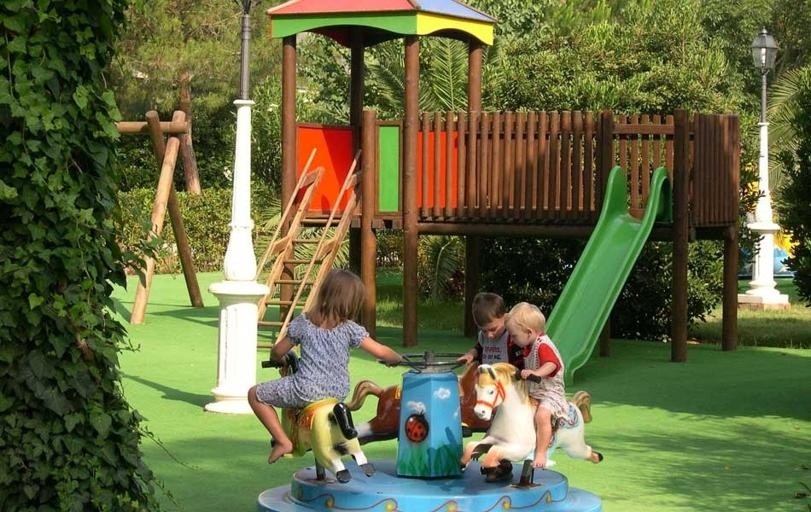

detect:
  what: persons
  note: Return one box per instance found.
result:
[456,293,523,370]
[504,301,572,469]
[248,268,401,463]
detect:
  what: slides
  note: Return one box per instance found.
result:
[543,165,668,386]
